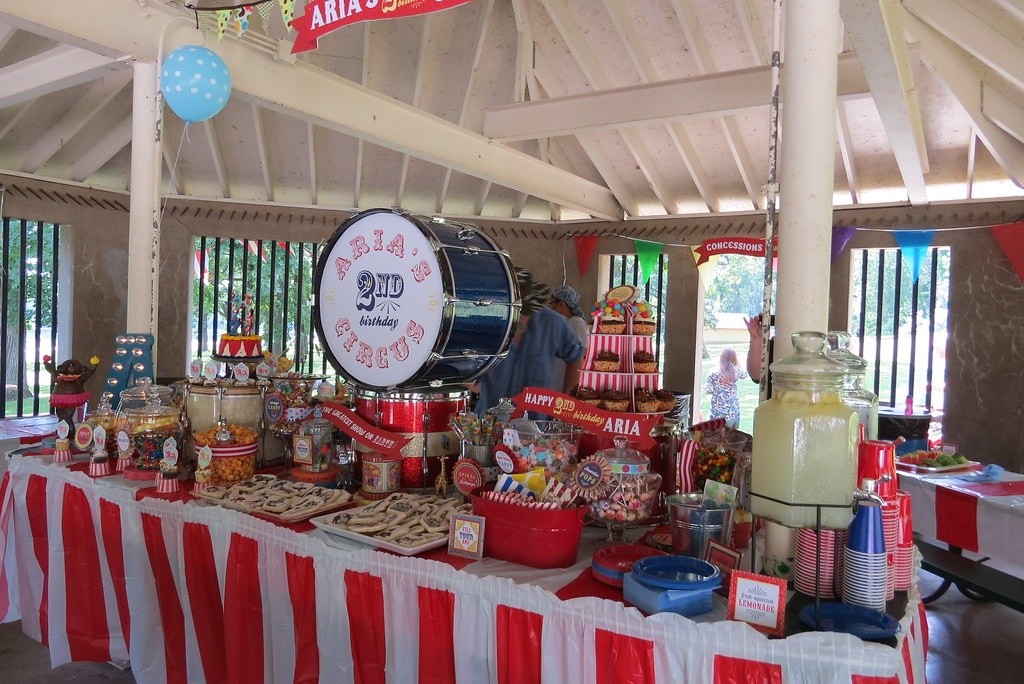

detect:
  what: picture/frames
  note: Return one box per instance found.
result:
[704,538,744,599]
[445,514,487,562]
[726,567,788,638]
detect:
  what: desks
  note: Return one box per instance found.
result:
[895,466,1024,605]
[0,452,926,684]
[1,412,63,473]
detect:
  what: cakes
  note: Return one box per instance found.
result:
[216,310,263,357]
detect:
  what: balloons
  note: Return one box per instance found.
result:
[159,45,232,123]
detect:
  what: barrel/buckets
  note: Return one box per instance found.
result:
[470,484,596,569]
[664,493,734,562]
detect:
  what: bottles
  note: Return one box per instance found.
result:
[301,405,335,473]
[587,436,662,530]
[85,377,185,469]
[750,332,879,535]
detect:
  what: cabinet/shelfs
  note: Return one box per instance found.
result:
[574,313,671,414]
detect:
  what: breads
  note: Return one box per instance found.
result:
[203,474,474,550]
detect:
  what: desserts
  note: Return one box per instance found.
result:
[189,350,294,387]
[570,321,678,412]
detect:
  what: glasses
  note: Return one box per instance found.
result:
[547,299,561,305]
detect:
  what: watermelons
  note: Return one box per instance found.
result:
[899,449,969,467]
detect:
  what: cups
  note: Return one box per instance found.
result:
[794,438,916,615]
[680,430,746,492]
[502,420,586,483]
[463,441,503,501]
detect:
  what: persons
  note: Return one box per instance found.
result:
[545,284,589,360]
[705,347,750,431]
[460,302,586,432]
[40,355,99,440]
[743,305,776,404]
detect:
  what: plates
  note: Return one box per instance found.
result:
[591,542,723,589]
[799,600,902,639]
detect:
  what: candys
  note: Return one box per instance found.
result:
[84,425,183,470]
[263,386,311,436]
[448,412,663,521]
[691,446,737,489]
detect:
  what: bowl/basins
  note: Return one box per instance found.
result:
[880,409,933,438]
[568,320,676,411]
